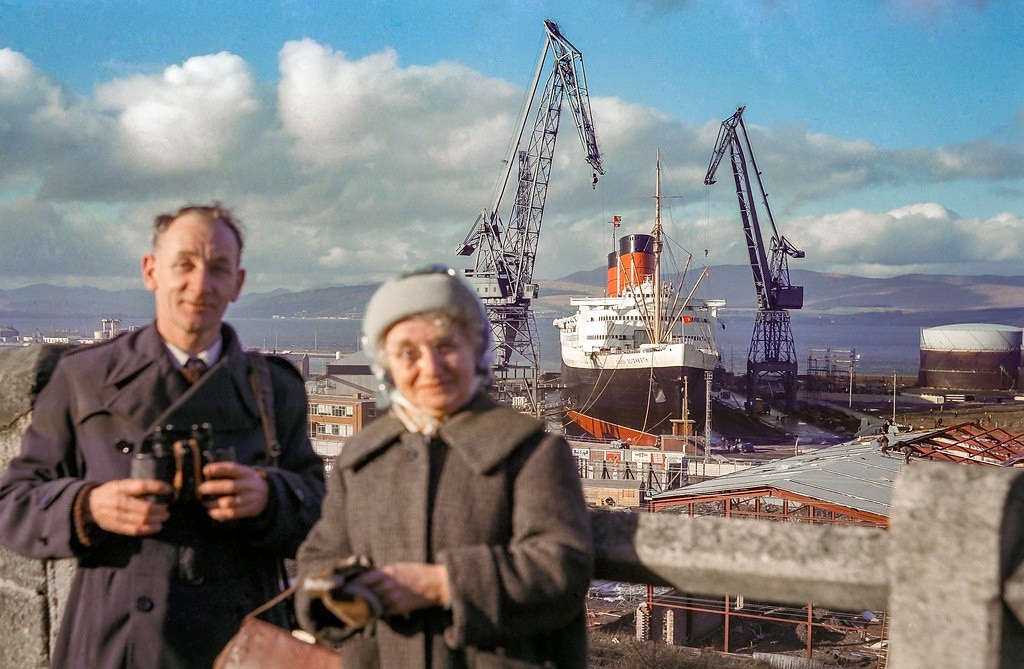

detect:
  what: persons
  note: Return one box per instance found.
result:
[877,409,992,465]
[296,263,597,668]
[0,199,328,669]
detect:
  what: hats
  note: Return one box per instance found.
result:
[363,262,493,372]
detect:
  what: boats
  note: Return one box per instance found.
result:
[551,149,727,446]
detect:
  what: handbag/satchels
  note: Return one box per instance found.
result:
[211,572,344,669]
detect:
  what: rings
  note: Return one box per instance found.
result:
[234,494,240,508]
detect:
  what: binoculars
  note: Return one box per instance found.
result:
[129,423,237,528]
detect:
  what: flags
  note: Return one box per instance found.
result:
[682,316,693,323]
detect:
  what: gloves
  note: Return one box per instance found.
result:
[298,555,376,634]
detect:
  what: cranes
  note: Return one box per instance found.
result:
[454,19,606,429]
[705,105,806,419]
